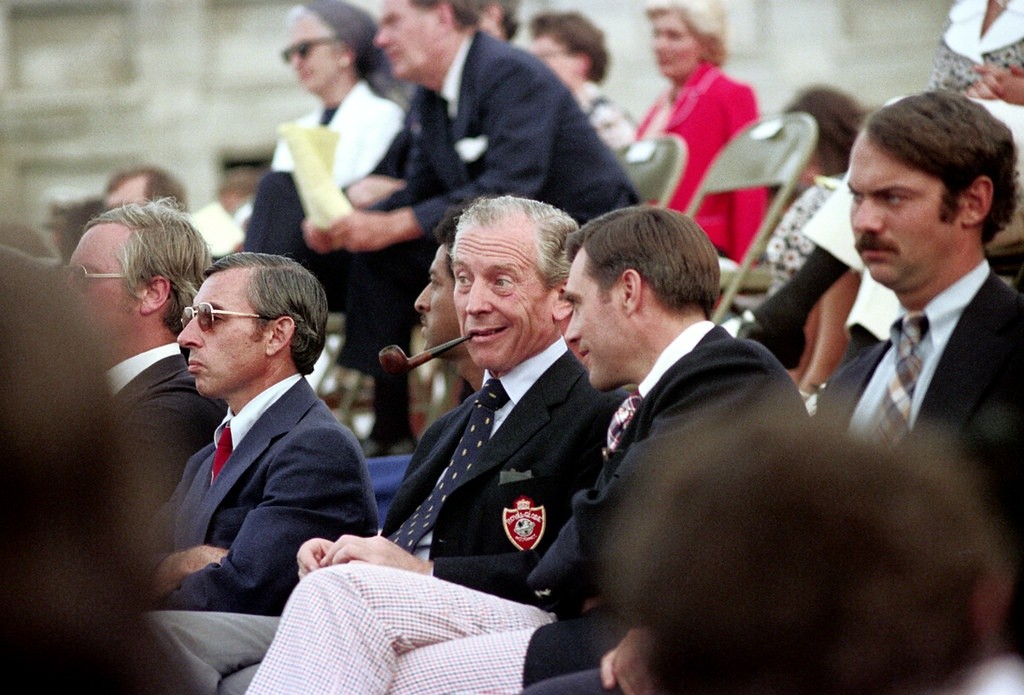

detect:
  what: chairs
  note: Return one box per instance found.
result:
[310,110,818,451]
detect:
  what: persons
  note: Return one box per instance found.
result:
[608,402,1024,695]
[139,195,630,695]
[0,204,197,694]
[241,205,808,695]
[29,0,1024,657]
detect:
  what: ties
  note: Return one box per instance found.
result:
[607,391,644,453]
[866,312,928,444]
[211,422,233,484]
[385,379,510,554]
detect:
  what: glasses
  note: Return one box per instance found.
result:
[180,302,277,332]
[281,37,334,63]
[62,264,126,284]
